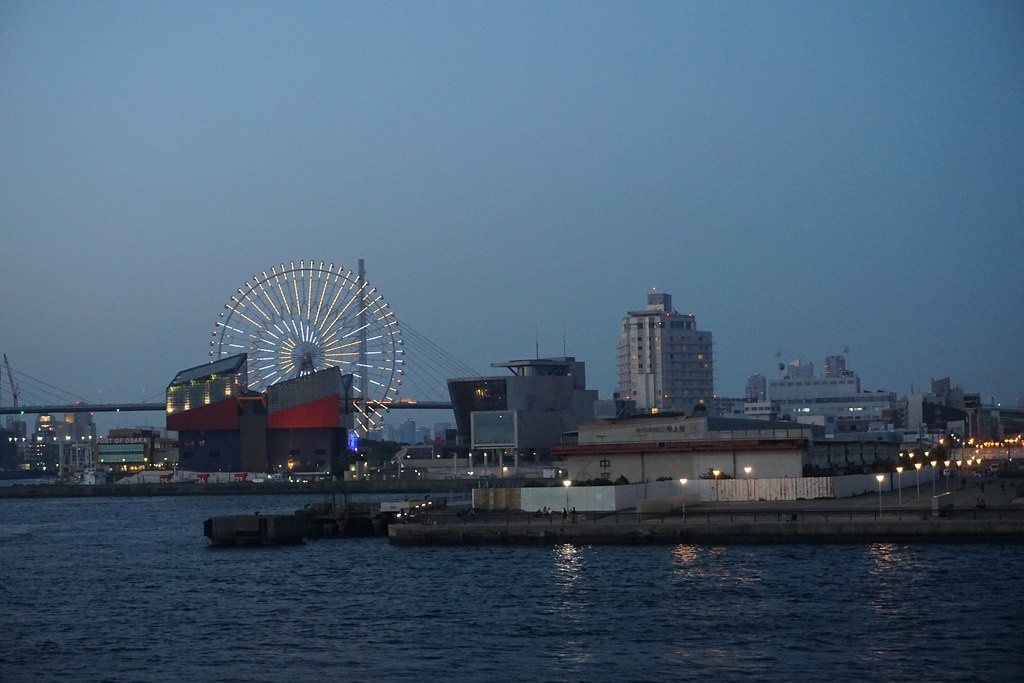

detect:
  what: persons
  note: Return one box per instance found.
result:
[469,506,575,516]
[972,468,991,478]
[976,498,985,511]
[1000,480,1006,495]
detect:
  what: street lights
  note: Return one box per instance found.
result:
[931,460,938,496]
[956,460,963,490]
[713,470,720,506]
[896,466,904,518]
[944,460,950,492]
[744,466,752,500]
[914,463,921,502]
[876,475,885,519]
[563,479,571,515]
[679,478,687,523]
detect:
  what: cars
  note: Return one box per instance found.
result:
[974,470,986,478]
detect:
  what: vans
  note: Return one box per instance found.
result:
[990,464,999,472]
